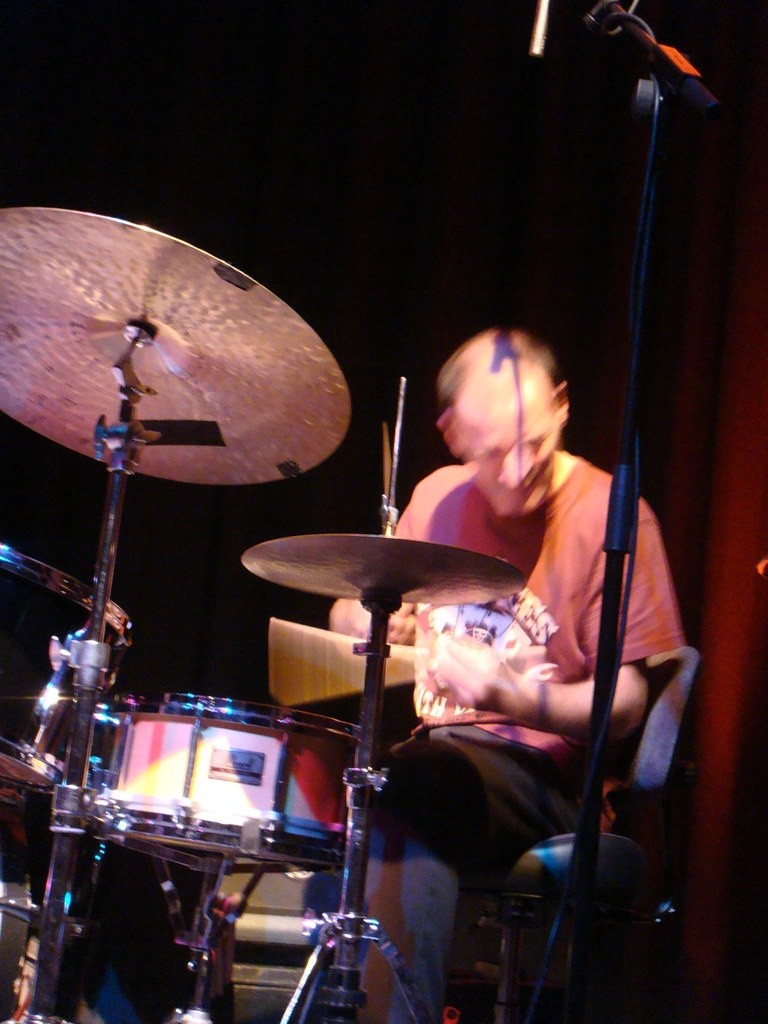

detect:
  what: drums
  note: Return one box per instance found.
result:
[199,841,487,1024]
[1,541,134,800]
[84,684,361,874]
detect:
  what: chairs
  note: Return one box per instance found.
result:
[459,645,702,1023]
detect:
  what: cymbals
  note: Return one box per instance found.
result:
[0,205,353,484]
[240,532,527,606]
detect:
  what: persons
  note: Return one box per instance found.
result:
[331,328,686,1024]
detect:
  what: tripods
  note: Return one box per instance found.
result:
[280,592,436,1024]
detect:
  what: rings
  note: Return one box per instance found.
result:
[436,682,448,690]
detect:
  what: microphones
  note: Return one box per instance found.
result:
[529,0,550,58]
